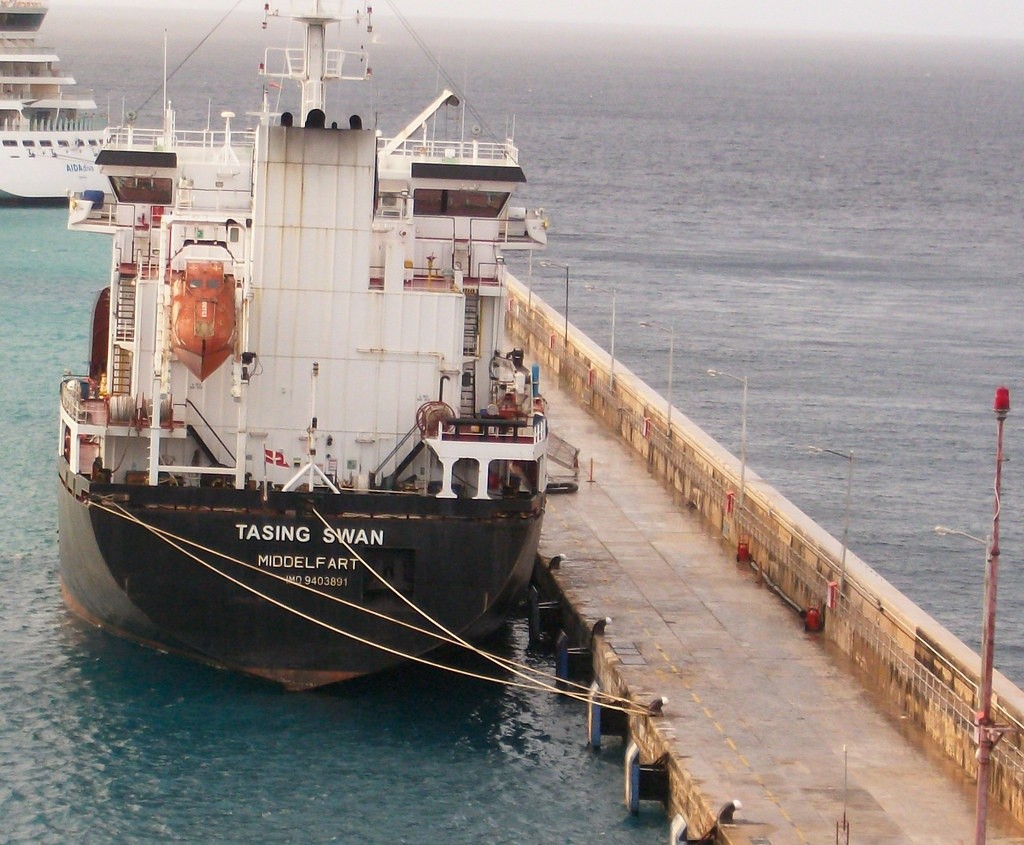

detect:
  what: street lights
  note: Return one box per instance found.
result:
[539,261,569,347]
[639,321,675,435]
[586,283,616,393]
[936,527,990,711]
[707,369,748,509]
[806,445,855,591]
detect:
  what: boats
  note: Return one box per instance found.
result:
[0,1,111,199]
[53,2,553,693]
